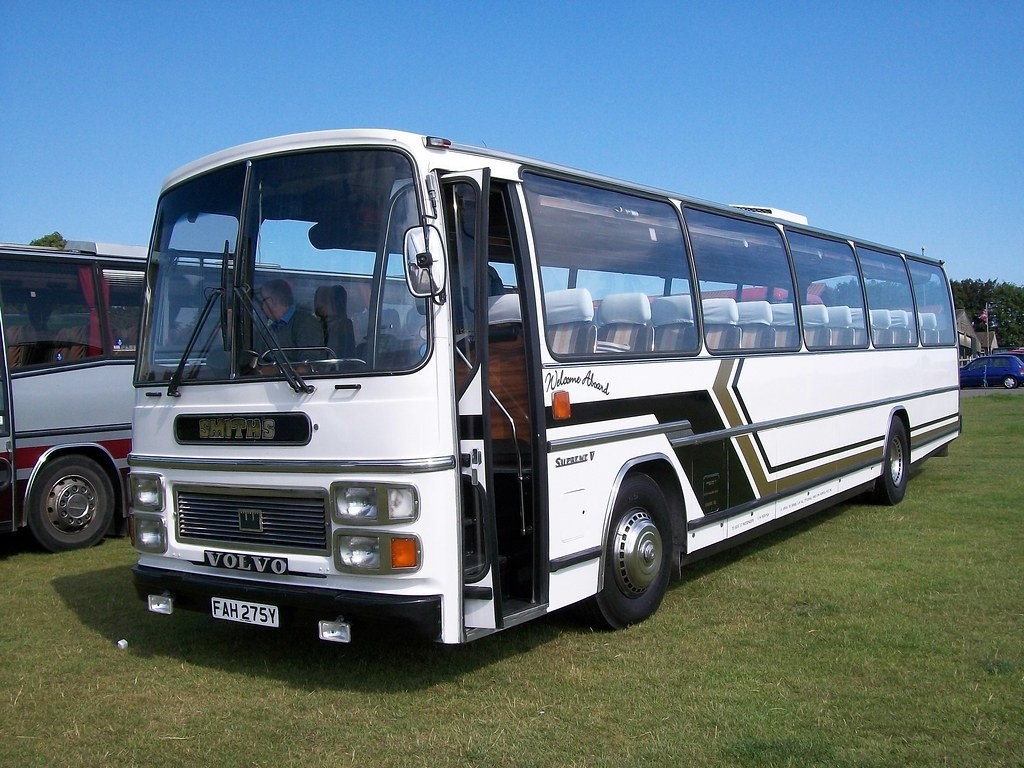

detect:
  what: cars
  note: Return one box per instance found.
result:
[991,346,1024,365]
[954,354,1024,388]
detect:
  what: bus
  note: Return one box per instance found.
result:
[129,129,963,673]
[1,238,523,553]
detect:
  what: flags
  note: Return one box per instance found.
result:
[979,309,987,324]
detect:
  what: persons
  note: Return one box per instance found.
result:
[247,279,329,382]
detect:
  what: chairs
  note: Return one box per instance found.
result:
[3,312,34,368]
[312,283,357,376]
[45,313,86,364]
[357,290,940,436]
